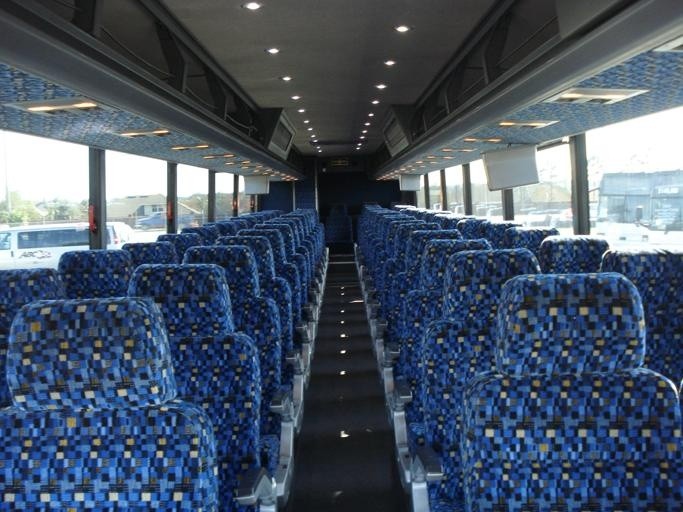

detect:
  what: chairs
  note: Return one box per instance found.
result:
[0,206,331,512]
[354,202,683,511]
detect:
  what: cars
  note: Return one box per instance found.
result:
[433,200,602,248]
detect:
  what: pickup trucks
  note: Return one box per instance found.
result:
[136,210,192,232]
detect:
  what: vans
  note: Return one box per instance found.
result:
[0,222,135,283]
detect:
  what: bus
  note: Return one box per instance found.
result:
[586,167,683,252]
